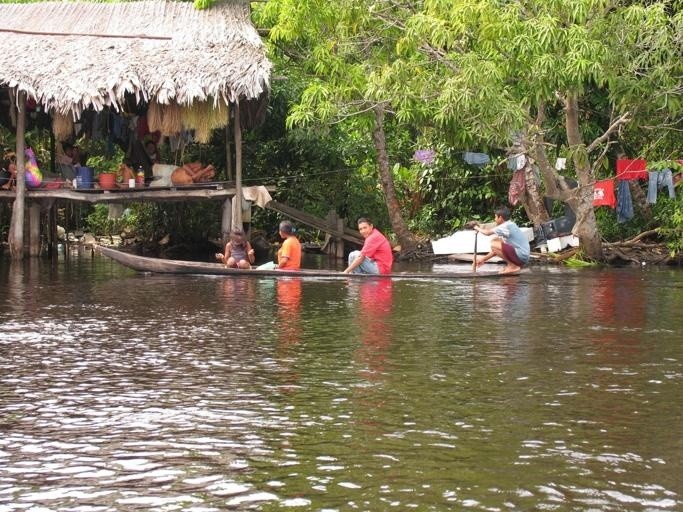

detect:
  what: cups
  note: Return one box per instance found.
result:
[128,178,135,188]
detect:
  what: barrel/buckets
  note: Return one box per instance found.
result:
[73,166,94,190]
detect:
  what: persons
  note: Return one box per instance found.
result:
[116,157,134,188]
[342,216,392,275]
[144,141,160,163]
[2,136,44,192]
[466,207,529,274]
[214,227,256,270]
[273,221,304,270]
[147,159,216,187]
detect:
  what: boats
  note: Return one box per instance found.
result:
[82,239,547,281]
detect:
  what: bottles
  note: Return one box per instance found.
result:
[72,177,77,190]
[136,164,145,184]
